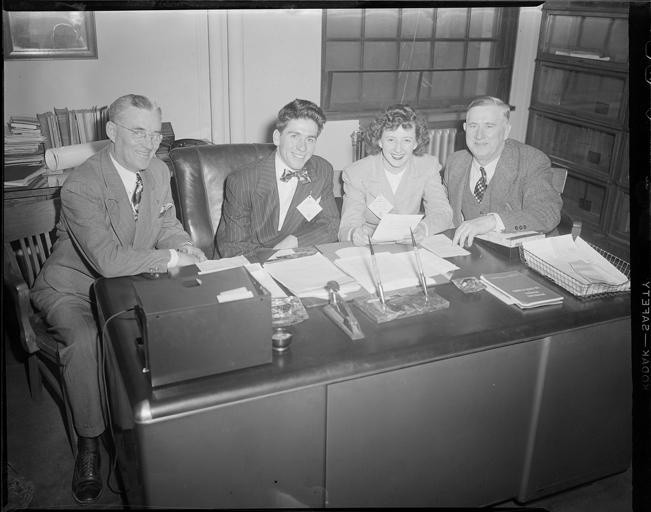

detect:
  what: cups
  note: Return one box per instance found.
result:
[272,315,293,351]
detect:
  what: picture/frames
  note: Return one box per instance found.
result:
[2,9,99,62]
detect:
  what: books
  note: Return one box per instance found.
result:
[4,116,49,169]
[478,271,564,309]
[36,104,111,149]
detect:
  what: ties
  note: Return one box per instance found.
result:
[132,172,143,223]
[475,167,487,203]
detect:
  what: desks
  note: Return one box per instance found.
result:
[89,228,632,512]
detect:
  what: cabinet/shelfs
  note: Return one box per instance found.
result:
[526,1,631,263]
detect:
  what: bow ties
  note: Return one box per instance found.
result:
[280,169,311,184]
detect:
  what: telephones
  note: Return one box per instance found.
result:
[170,139,211,149]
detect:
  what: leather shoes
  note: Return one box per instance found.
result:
[72,449,104,503]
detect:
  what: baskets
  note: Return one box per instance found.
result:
[519,234,631,302]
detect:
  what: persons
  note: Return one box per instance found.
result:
[28,94,210,504]
[442,95,566,248]
[212,98,340,266]
[336,102,454,248]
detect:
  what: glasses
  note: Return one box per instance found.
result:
[113,121,163,144]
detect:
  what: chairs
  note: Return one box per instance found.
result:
[358,115,463,179]
[174,140,278,259]
[2,199,80,460]
